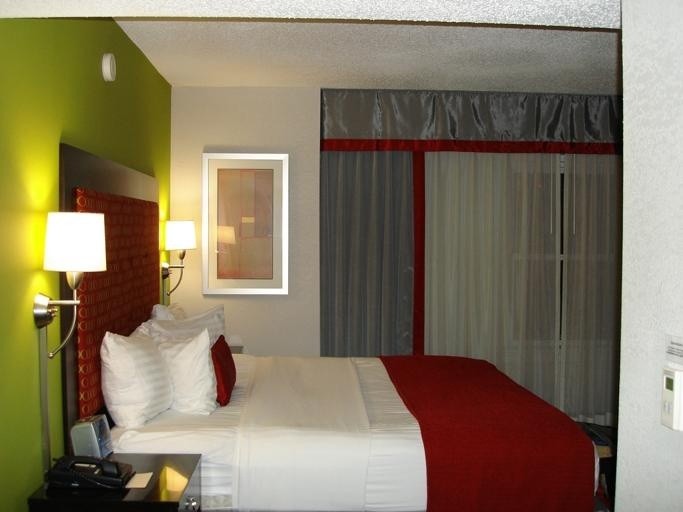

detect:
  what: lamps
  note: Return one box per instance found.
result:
[35,211,109,359]
[161,220,198,296]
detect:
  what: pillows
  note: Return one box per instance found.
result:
[98,304,238,428]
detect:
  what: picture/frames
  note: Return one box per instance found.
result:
[201,152,289,296]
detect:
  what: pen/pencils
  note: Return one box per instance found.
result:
[126,471,136,484]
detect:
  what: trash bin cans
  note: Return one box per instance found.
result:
[580,420,616,480]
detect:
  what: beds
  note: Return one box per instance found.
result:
[58,142,600,508]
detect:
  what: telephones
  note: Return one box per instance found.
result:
[48,455,133,488]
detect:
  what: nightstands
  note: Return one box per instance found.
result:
[27,452,202,511]
